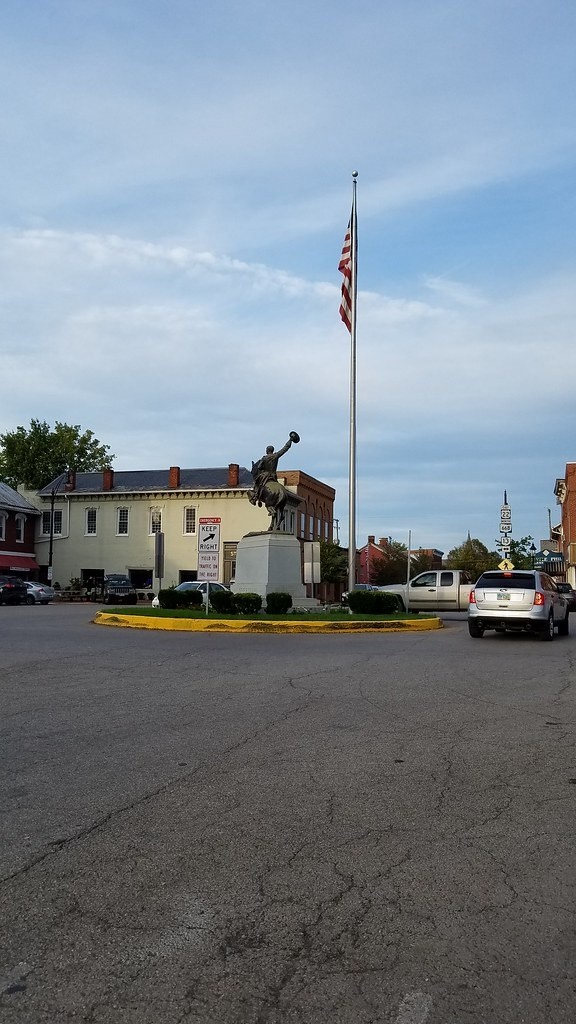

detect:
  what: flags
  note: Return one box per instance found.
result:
[338,214,353,334]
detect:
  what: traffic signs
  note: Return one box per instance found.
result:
[198,525,219,551]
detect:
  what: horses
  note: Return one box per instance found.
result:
[251,461,288,531]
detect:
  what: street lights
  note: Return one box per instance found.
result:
[47,468,71,586]
[531,543,537,570]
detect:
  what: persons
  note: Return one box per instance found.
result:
[246,438,292,506]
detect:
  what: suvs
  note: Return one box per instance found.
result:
[91,574,137,605]
[0,576,27,605]
[468,570,576,641]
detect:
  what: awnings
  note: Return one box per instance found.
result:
[0,555,40,571]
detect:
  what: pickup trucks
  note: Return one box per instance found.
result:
[378,569,475,614]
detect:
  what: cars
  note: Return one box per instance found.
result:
[152,581,230,609]
[342,584,378,605]
[24,581,54,604]
